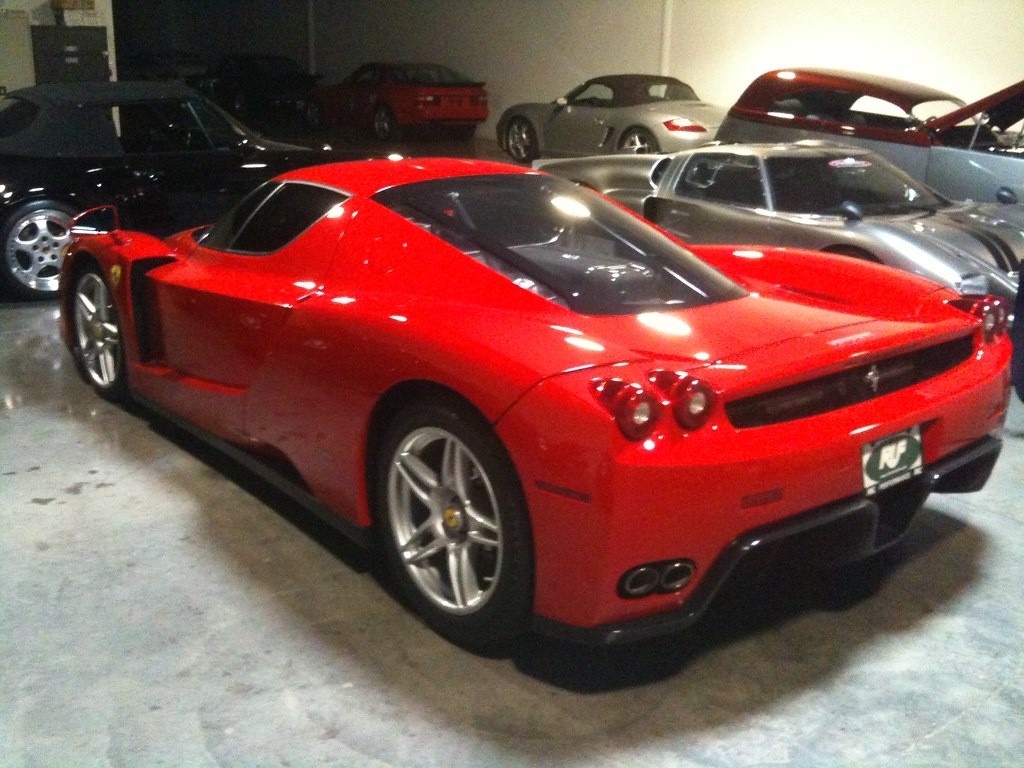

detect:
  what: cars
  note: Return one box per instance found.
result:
[495,69,1024,296]
[309,63,488,143]
[59,157,1014,646]
[0,81,316,300]
